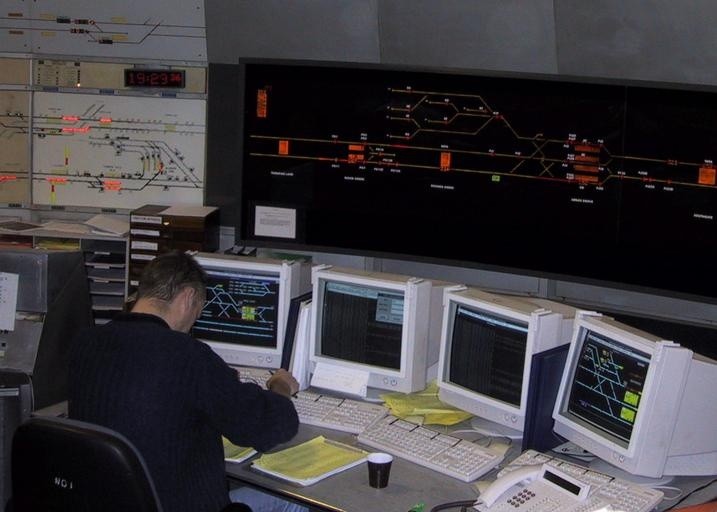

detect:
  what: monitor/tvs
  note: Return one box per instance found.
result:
[552,316,717,486]
[185,252,313,369]
[436,287,589,439]
[311,264,459,402]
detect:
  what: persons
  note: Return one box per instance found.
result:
[64,253,298,512]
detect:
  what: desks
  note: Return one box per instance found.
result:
[31,374,716,512]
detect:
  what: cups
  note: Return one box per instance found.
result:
[365,452,393,488]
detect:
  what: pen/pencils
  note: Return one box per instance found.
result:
[268,370,298,399]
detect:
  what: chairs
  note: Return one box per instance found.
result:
[8,410,168,509]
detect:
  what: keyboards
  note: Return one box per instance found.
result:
[358,414,505,482]
[497,449,665,512]
[239,370,277,391]
[291,392,389,433]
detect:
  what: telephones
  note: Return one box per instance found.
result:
[472,463,591,512]
